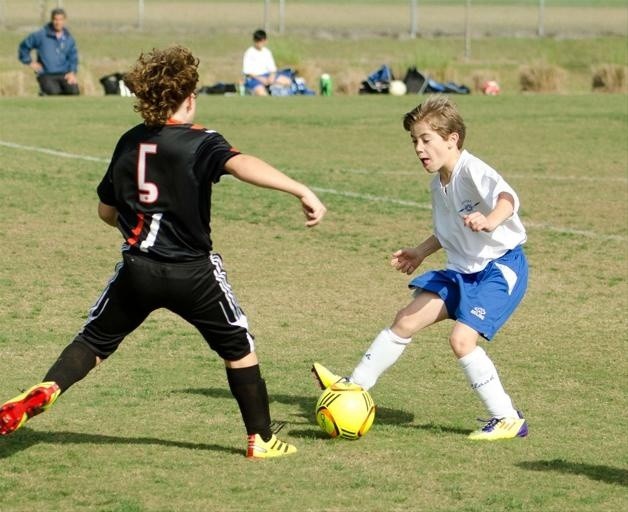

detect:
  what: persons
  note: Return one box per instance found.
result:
[240,29,292,98]
[1,44,329,462]
[16,8,82,97]
[308,92,532,442]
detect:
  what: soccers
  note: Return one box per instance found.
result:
[316,382,376,440]
[483,81,499,95]
[388,80,406,95]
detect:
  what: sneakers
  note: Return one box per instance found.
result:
[467,409,530,442]
[0,381,61,437]
[309,362,342,393]
[246,433,297,462]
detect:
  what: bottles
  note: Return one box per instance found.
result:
[235,80,245,97]
[320,75,331,96]
